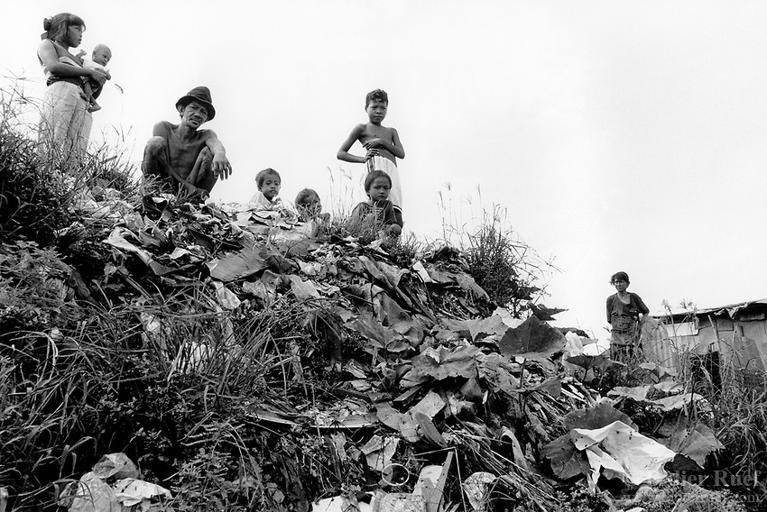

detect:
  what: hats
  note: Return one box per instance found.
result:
[175,86,216,121]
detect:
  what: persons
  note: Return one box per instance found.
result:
[140,86,232,209]
[295,188,331,224]
[73,44,112,113]
[35,12,105,152]
[605,272,648,362]
[349,170,404,245]
[246,168,284,207]
[335,89,404,237]
